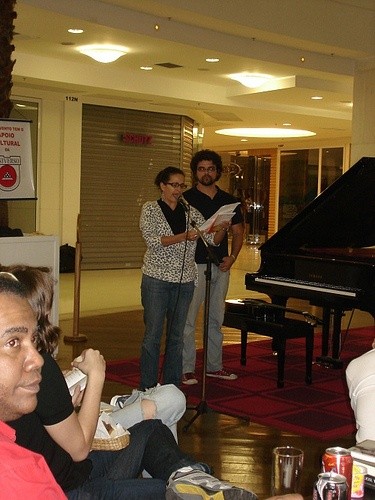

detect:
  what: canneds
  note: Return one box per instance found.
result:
[312,471,350,500]
[322,447,353,500]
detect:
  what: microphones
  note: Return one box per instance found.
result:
[177,194,189,205]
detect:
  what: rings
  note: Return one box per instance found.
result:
[194,235,197,238]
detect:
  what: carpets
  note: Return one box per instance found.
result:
[106,327,375,440]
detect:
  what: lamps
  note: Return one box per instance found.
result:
[79,48,127,63]
[236,74,270,89]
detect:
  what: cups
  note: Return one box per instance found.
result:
[270,446,304,496]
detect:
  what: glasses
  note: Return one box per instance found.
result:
[167,182,188,190]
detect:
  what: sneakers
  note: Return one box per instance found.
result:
[183,373,198,385]
[205,369,238,380]
[164,469,258,500]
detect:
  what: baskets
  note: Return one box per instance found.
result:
[92,433,130,451]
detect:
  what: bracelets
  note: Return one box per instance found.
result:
[231,255,235,258]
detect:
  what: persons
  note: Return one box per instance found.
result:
[182,150,244,384]
[99,383,187,478]
[0,266,260,500]
[139,167,232,391]
[345,340,375,451]
[0,276,69,500]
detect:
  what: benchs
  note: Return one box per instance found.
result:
[222,298,316,388]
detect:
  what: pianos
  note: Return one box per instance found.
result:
[243,155,375,371]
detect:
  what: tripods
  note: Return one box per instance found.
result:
[180,205,250,433]
